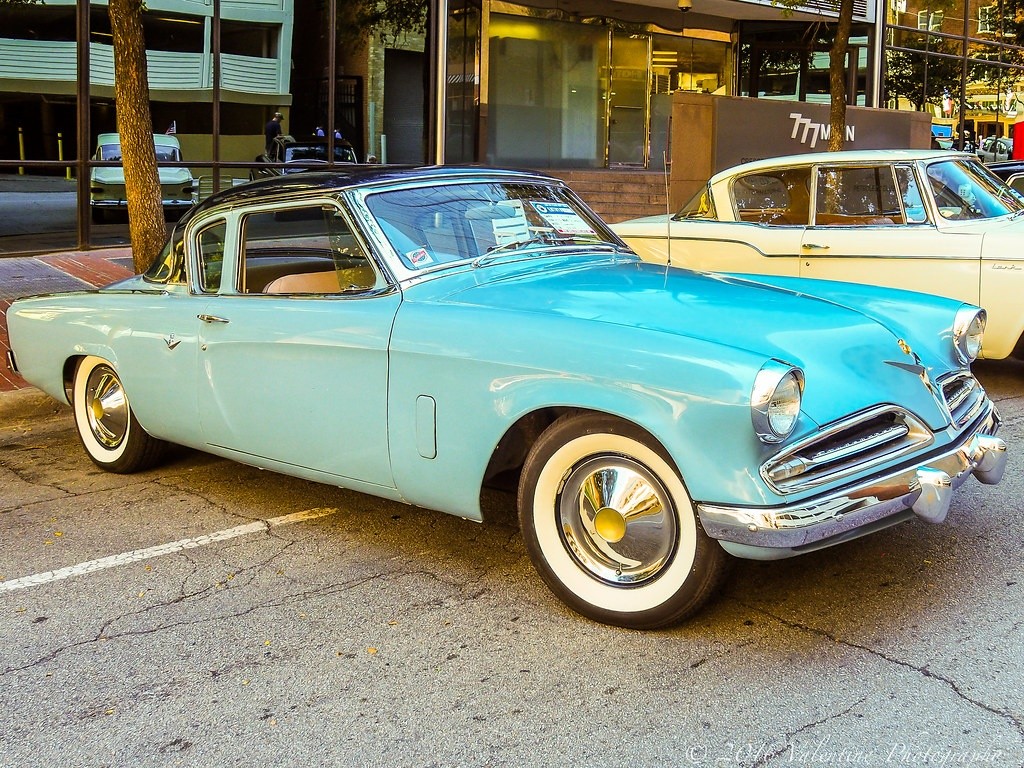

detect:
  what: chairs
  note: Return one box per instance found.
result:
[865,218,895,224]
[830,221,865,225]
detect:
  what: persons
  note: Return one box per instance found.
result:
[930,131,941,150]
[264,105,284,160]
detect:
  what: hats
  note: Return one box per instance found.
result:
[274,112,284,120]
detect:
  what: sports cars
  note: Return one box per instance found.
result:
[5,165,1008,631]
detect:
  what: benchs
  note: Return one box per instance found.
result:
[262,266,376,294]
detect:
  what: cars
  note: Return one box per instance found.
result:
[976,135,1024,199]
[249,133,357,183]
[606,150,1024,365]
[88,132,196,224]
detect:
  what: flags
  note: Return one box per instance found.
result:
[164,121,175,134]
[942,87,952,114]
